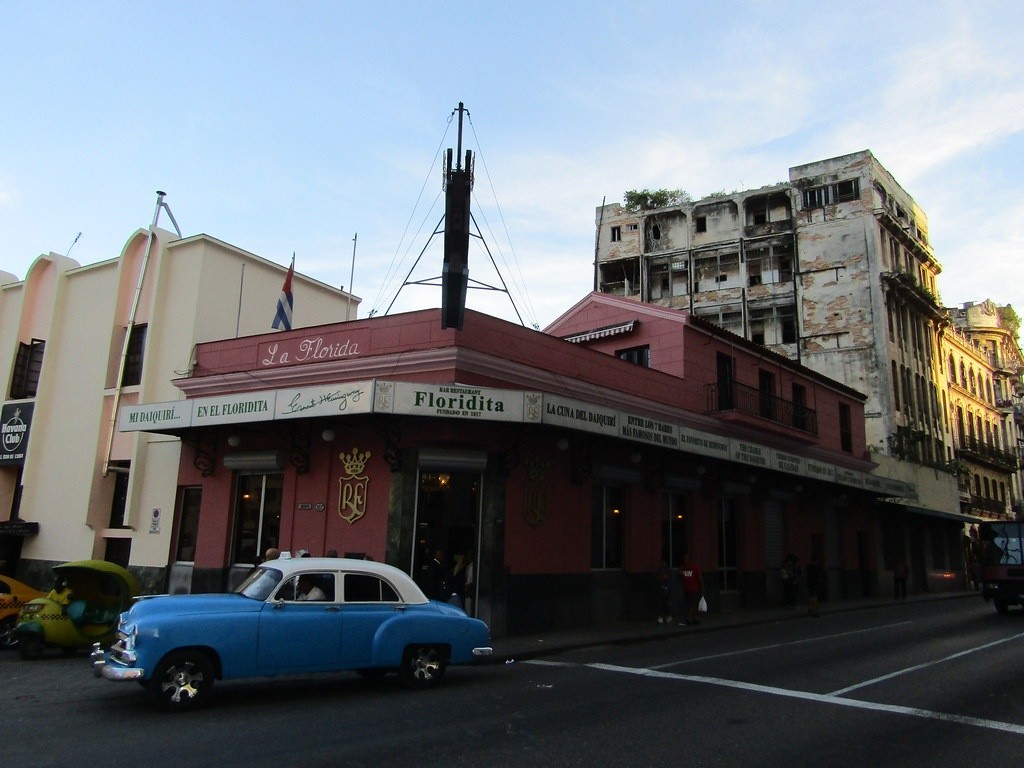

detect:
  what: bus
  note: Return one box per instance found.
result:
[977,520,1024,613]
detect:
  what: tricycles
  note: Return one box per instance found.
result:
[13,558,144,659]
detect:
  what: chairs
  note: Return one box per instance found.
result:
[65,599,90,622]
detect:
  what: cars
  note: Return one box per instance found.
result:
[89,550,493,710]
[0,574,51,649]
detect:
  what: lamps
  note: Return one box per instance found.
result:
[321,425,335,442]
[227,433,241,447]
[437,474,450,488]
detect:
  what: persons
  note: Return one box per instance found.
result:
[783,552,804,609]
[295,575,326,601]
[804,555,822,618]
[676,553,703,627]
[247,556,265,579]
[655,567,673,624]
[446,549,468,611]
[427,549,449,602]
[266,548,280,562]
[893,557,909,600]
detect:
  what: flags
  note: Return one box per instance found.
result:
[271,262,294,331]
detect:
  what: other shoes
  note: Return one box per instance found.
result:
[894,594,899,599]
[684,619,701,625]
[901,593,906,600]
[808,609,821,618]
